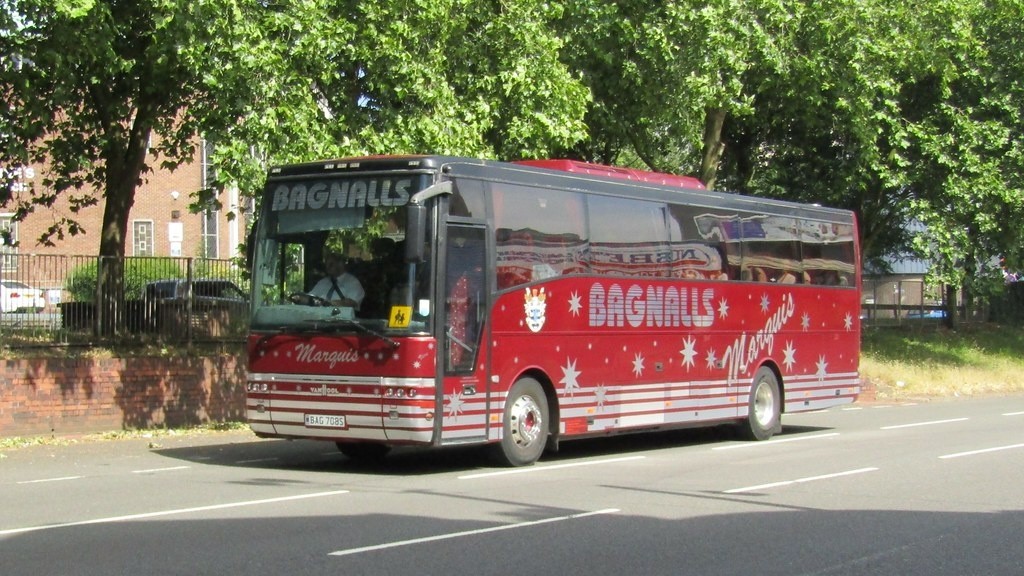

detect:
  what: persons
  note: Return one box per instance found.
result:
[681,252,812,284]
[290,253,366,311]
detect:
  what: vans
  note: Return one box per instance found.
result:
[137,277,247,326]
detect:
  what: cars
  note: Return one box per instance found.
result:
[0,280,46,314]
[905,301,945,320]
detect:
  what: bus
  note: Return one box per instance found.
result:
[236,155,863,469]
